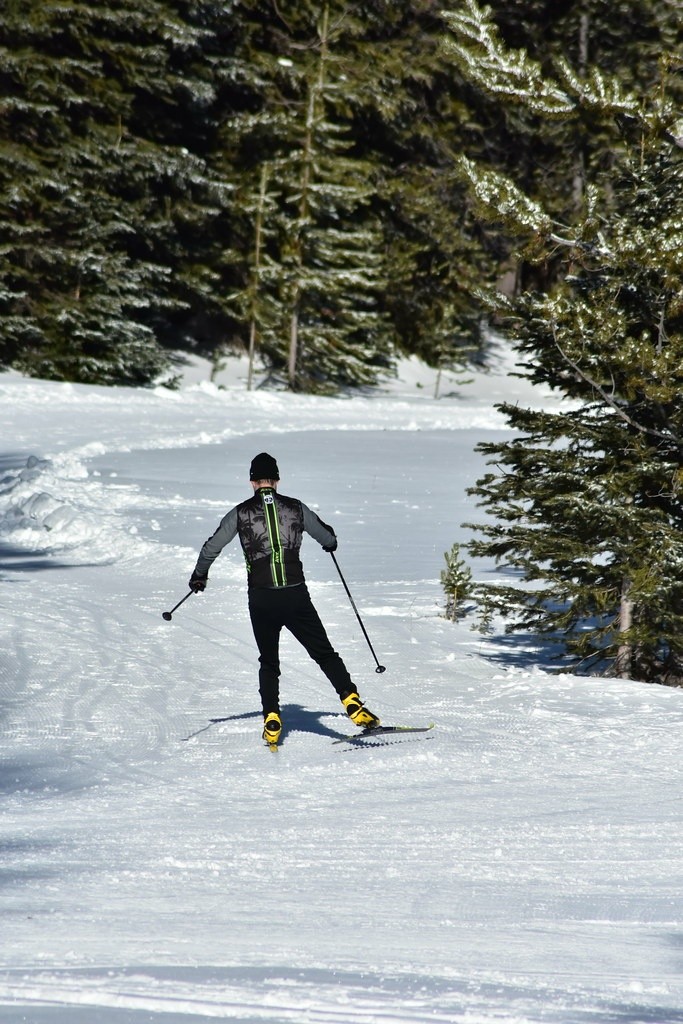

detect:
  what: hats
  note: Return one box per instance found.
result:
[249,453,279,481]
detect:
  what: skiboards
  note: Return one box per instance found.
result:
[268,724,433,754]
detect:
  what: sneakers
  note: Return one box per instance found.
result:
[262,712,283,744]
[341,693,380,728]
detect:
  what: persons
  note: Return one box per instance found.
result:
[187,452,379,746]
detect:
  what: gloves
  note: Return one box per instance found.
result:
[322,539,337,552]
[189,570,209,593]
